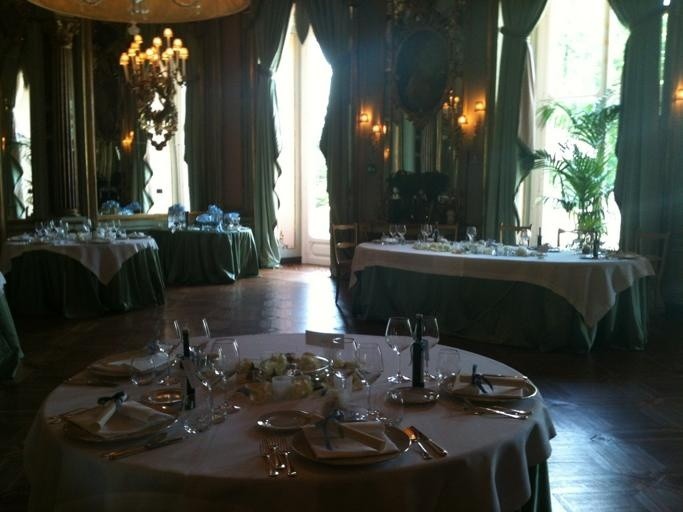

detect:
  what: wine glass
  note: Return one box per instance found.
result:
[170,314,440,424]
[384,223,531,250]
[38,219,122,241]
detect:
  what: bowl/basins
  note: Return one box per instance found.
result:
[255,354,330,389]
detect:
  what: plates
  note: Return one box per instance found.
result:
[139,388,184,406]
[67,406,173,442]
[436,375,536,402]
[291,431,412,465]
[88,357,173,379]
[255,408,311,432]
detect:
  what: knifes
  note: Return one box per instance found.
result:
[410,426,448,459]
[108,435,187,459]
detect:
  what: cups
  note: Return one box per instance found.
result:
[373,386,405,428]
[128,356,155,384]
[434,345,461,377]
[270,374,311,400]
[180,392,212,434]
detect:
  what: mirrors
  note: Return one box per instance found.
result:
[80,21,205,223]
[386,5,458,131]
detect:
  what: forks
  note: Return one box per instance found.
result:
[259,435,295,480]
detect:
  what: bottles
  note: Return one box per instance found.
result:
[536,227,542,245]
[175,328,196,412]
[409,312,428,388]
[433,220,440,243]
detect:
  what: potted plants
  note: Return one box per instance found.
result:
[385,168,450,226]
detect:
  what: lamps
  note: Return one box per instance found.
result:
[119,28,188,151]
